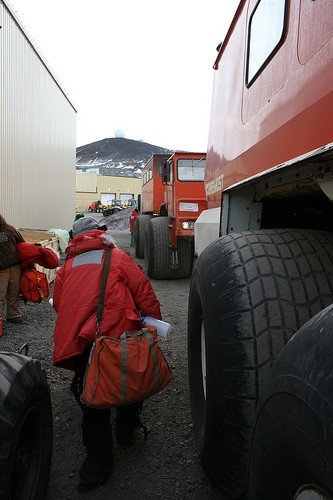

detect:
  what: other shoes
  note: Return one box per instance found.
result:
[115,424,149,445]
[76,466,114,491]
[6,318,23,323]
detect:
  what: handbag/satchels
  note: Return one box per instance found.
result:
[80,328,173,408]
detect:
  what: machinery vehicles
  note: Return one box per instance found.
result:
[133,150,208,280]
[102,198,123,218]
[88,199,106,213]
[121,198,137,210]
[186,0,333,500]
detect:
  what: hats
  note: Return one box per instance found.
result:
[72,217,99,236]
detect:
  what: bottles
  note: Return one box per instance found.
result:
[140,316,172,337]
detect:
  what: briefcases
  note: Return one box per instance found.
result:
[20,269,50,304]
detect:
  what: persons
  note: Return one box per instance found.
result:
[129,209,139,247]
[0,214,35,323]
[53,217,162,493]
[69,214,85,242]
